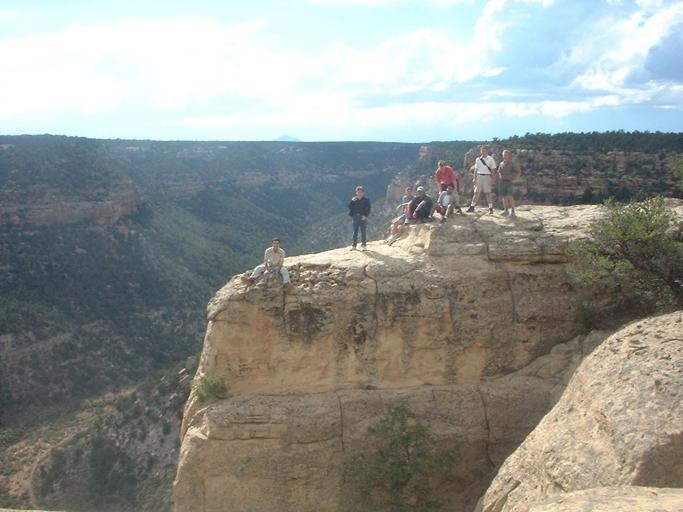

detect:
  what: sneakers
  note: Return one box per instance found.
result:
[466,206,474,212]
[499,209,515,216]
[489,208,493,214]
[241,277,252,285]
[350,245,367,251]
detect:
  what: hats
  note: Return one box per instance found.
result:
[417,186,424,192]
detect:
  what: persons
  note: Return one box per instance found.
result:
[240,237,293,290]
[465,146,497,215]
[394,186,414,214]
[427,183,468,223]
[434,160,460,214]
[346,185,372,252]
[384,205,409,246]
[405,186,431,221]
[496,149,520,218]
